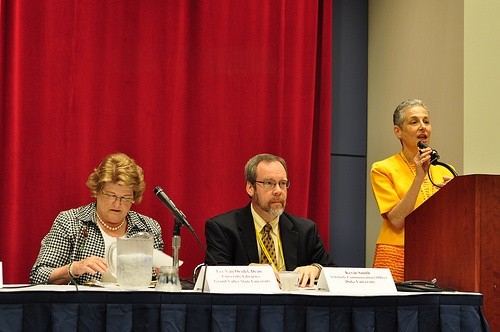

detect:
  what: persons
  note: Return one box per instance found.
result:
[29,152,165,284]
[204,154,334,289]
[370,99,455,282]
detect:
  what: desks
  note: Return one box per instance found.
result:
[0,283,490,332]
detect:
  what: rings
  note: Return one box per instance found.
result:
[420,160,423,164]
[419,149,422,155]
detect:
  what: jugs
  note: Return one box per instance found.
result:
[106,232,155,289]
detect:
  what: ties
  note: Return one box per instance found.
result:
[261,223,277,268]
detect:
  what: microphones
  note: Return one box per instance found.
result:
[417,141,439,158]
[153,186,195,232]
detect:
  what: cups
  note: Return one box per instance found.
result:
[156,266,181,291]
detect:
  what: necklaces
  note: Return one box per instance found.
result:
[96,213,125,231]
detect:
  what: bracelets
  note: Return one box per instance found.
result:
[68,261,79,278]
[312,263,321,271]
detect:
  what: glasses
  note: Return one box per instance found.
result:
[100,186,136,206]
[250,179,290,190]
